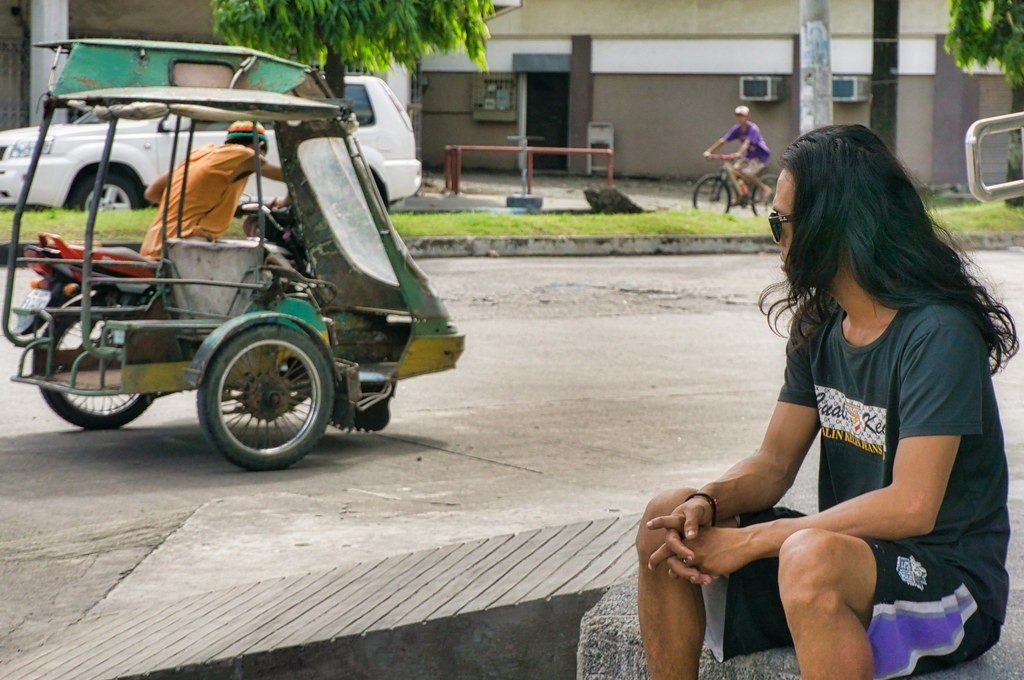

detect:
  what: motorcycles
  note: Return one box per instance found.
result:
[13,198,301,433]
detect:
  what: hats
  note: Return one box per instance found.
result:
[225,120,268,145]
[735,106,749,115]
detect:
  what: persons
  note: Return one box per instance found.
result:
[635,125,1020,680]
[704,105,772,205]
[140,119,286,265]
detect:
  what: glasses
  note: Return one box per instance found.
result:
[768,211,793,242]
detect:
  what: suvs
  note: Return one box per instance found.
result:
[0,67,421,215]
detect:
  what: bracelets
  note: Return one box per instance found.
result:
[685,493,717,528]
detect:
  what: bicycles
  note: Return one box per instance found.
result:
[692,152,777,220]
